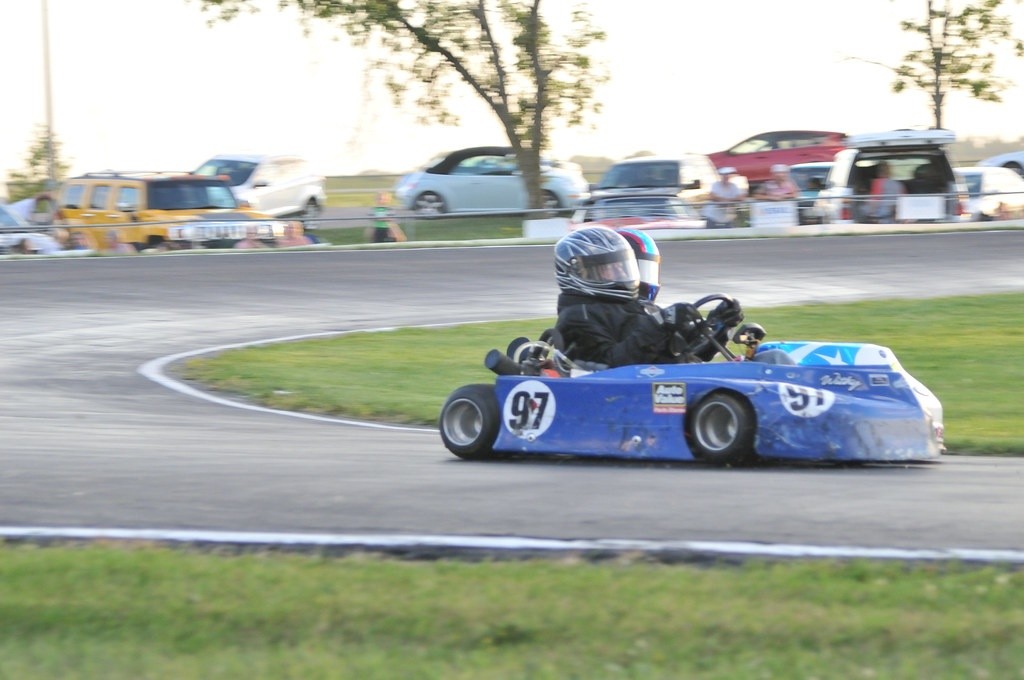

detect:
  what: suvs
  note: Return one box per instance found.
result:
[51,167,291,255]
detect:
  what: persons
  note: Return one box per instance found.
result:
[752,165,797,203]
[809,178,825,190]
[706,166,743,229]
[867,162,905,223]
[105,230,135,253]
[277,222,312,248]
[42,230,69,252]
[615,228,662,303]
[18,239,31,254]
[555,227,745,369]
[366,192,403,243]
[69,231,89,250]
[234,226,267,249]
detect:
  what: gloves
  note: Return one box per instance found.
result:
[648,303,703,334]
[707,298,745,330]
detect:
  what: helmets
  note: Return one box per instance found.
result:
[615,228,662,302]
[554,227,640,301]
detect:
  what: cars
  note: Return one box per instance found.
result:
[565,128,1024,228]
[394,144,592,221]
[180,152,328,222]
[706,128,848,183]
[0,198,63,256]
